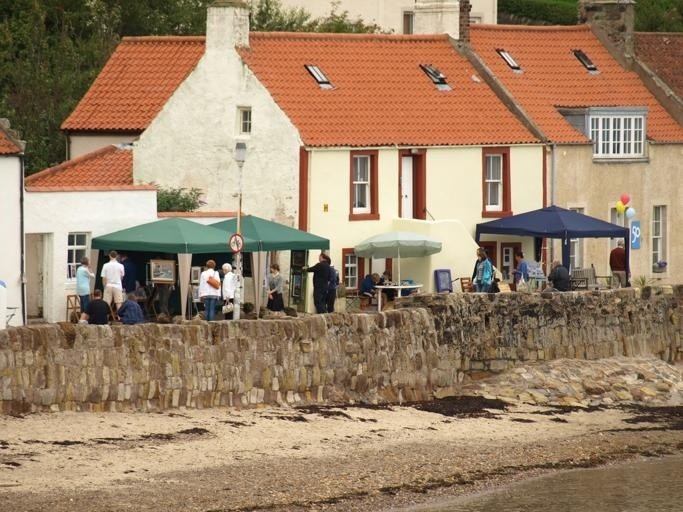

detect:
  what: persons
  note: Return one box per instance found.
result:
[73,250,149,326]
[197,259,221,321]
[471,248,572,293]
[607,237,626,290]
[300,251,330,314]
[220,261,236,320]
[263,263,285,319]
[328,262,339,312]
[357,271,396,308]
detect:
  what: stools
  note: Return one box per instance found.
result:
[4,306,19,326]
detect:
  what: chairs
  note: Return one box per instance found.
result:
[66,287,202,322]
[458,263,613,294]
[348,264,422,312]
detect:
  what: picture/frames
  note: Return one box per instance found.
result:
[150,259,176,282]
[190,266,200,284]
[291,272,303,299]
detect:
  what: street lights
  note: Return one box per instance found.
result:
[229,141,246,322]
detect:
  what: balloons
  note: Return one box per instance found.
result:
[615,192,635,218]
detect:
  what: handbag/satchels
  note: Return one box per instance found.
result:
[516,279,525,291]
[222,303,233,314]
[207,276,220,289]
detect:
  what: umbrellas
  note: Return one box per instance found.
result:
[352,230,442,298]
